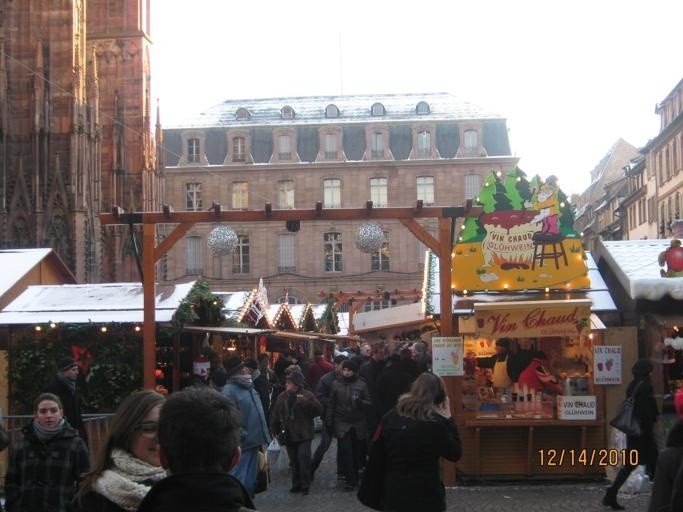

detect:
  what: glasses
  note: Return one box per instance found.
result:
[134,422,159,440]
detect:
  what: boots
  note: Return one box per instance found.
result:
[603,488,625,510]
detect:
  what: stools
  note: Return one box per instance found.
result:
[532,240,568,271]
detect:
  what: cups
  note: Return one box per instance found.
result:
[512,383,541,412]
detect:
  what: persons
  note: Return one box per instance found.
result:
[601,359,683,512]
[356,372,461,512]
[463,338,564,401]
[273,340,429,494]
[1,354,271,511]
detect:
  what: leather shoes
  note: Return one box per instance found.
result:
[337,474,354,490]
[289,487,309,495]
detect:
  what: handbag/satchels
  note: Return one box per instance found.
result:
[609,381,645,439]
[276,416,289,446]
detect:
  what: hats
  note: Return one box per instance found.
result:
[496,338,510,346]
[223,357,257,373]
[286,371,305,387]
[631,359,654,373]
[342,358,358,372]
[56,357,77,372]
